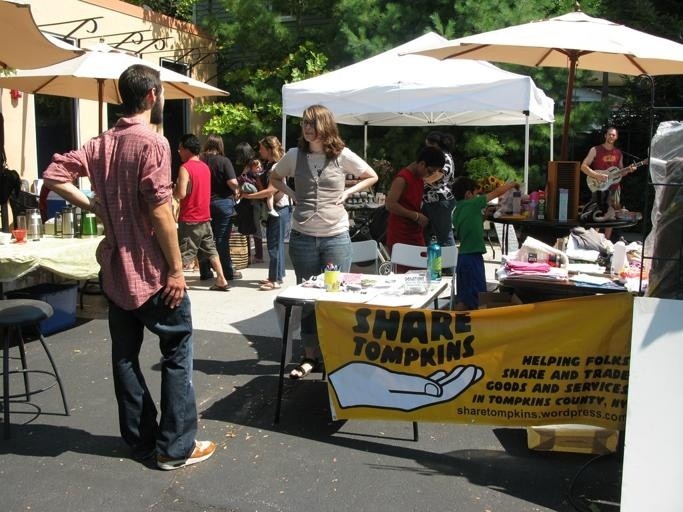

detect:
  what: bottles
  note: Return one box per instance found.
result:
[425,236,442,282]
[528,199,536,218]
[16,206,96,238]
[538,199,545,219]
[511,191,522,217]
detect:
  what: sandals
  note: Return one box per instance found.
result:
[290,358,319,378]
[260,282,281,291]
[259,279,270,283]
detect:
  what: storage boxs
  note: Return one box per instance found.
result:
[2,282,78,338]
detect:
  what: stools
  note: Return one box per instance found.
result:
[0,298,69,447]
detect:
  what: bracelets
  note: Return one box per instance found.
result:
[414,212,419,222]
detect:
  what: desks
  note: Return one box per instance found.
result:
[495,267,629,305]
[345,203,391,263]
[481,206,638,250]
[272,271,448,443]
[0,232,107,312]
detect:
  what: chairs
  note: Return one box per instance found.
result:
[390,242,459,310]
[349,240,379,276]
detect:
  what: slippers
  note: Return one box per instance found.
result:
[210,284,231,291]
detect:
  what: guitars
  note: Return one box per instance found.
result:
[587,159,649,193]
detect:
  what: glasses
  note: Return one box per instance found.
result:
[299,120,313,128]
[426,167,432,176]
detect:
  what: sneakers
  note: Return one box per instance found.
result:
[231,272,242,279]
[156,439,217,470]
[208,271,214,278]
[267,208,279,217]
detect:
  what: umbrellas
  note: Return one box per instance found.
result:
[398,0,683,162]
[0,34,230,136]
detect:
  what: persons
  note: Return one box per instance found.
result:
[198,134,242,280]
[420,131,457,247]
[235,142,264,263]
[42,64,216,472]
[172,134,229,291]
[580,127,638,240]
[385,146,444,255]
[271,105,378,285]
[258,136,294,291]
[451,177,515,311]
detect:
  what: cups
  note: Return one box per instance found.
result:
[322,270,342,292]
[10,229,27,245]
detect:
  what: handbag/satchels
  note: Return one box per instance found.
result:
[234,197,257,235]
